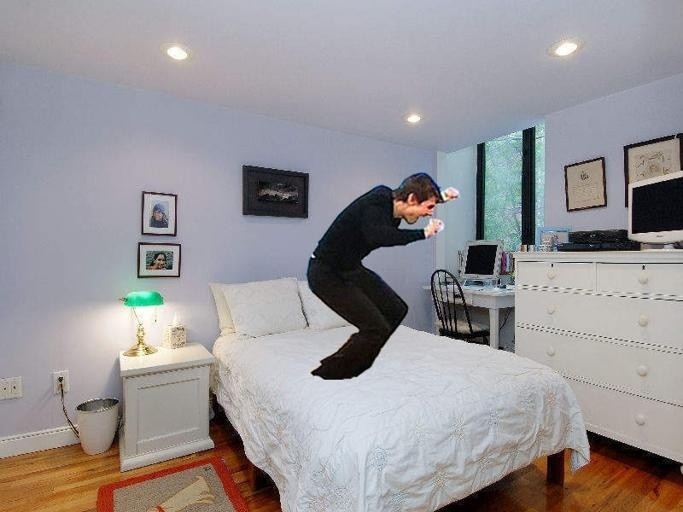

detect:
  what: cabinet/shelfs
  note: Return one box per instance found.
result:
[510,248,683,465]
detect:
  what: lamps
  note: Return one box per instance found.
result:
[117,290,167,357]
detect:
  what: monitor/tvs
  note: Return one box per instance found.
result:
[628,170,683,249]
[460,240,503,290]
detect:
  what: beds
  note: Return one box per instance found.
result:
[208,322,594,512]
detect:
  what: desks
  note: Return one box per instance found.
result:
[421,284,515,349]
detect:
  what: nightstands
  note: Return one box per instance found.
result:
[115,342,216,474]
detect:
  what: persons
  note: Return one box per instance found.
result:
[146,252,166,270]
[149,202,167,227]
[305,171,459,380]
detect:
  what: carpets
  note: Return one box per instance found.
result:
[94,451,252,512]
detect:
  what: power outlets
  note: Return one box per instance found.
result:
[52,369,70,396]
[0,374,24,402]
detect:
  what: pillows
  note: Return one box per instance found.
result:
[219,274,307,340]
[295,277,352,334]
[207,279,235,337]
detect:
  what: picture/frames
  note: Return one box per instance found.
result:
[240,163,309,220]
[622,132,682,209]
[136,241,181,279]
[140,190,177,237]
[563,156,608,212]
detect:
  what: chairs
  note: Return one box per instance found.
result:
[430,269,489,346]
